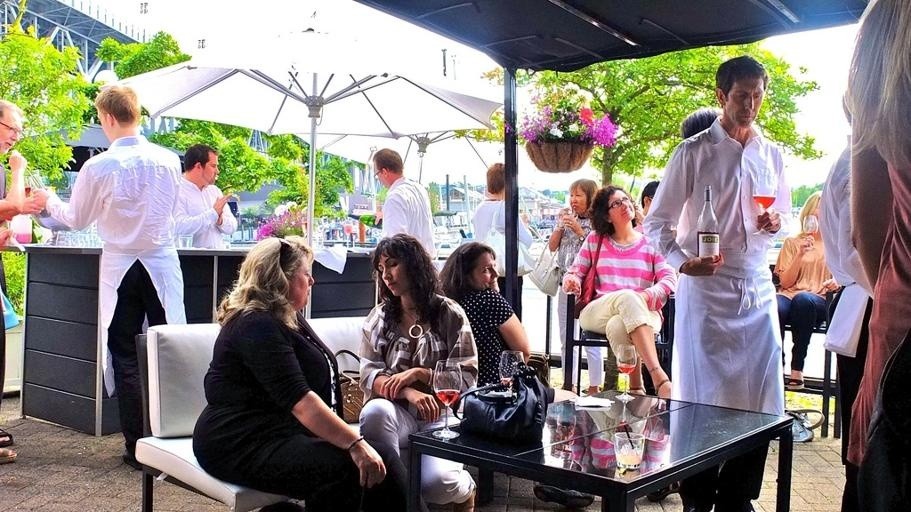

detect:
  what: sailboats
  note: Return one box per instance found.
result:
[433,172,558,260]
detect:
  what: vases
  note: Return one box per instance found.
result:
[526,139,596,174]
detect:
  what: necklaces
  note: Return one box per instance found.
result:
[400,308,424,339]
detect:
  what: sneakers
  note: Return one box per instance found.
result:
[533,481,595,508]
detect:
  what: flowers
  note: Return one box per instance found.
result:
[505,94,618,149]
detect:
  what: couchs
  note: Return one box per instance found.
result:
[130,315,499,511]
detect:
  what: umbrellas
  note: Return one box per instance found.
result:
[291,131,499,183]
[112,7,507,315]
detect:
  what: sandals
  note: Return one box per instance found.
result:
[0,428,19,464]
[789,375,806,390]
[628,377,672,401]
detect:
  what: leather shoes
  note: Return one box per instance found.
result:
[124,448,144,471]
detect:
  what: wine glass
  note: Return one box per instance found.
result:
[615,344,638,400]
[343,221,352,249]
[802,215,819,251]
[752,174,776,237]
[432,359,464,441]
[617,400,634,431]
[565,253,581,296]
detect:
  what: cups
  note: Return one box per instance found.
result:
[562,209,579,230]
[178,235,193,249]
[23,169,48,200]
[54,223,104,248]
[520,211,529,225]
[498,350,525,383]
[614,432,647,470]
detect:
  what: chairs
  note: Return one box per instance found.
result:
[564,289,677,397]
[768,263,835,441]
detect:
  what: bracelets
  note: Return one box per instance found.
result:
[426,367,433,388]
[346,436,364,451]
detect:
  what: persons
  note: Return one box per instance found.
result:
[562,185,677,400]
[179,144,238,251]
[548,180,604,395]
[642,54,789,512]
[774,190,843,391]
[848,1,910,512]
[0,99,44,464]
[359,233,479,511]
[440,243,595,509]
[470,163,532,328]
[192,237,429,511]
[816,92,875,512]
[341,150,435,263]
[680,107,723,140]
[33,85,187,469]
[640,181,660,215]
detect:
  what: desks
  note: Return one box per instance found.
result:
[406,389,795,511]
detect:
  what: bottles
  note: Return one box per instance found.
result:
[347,213,382,230]
[10,218,33,244]
[696,184,721,258]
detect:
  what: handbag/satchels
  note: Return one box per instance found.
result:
[332,348,365,424]
[785,408,826,443]
[529,241,562,297]
[451,365,548,440]
[482,200,536,277]
[0,285,21,330]
[573,231,605,319]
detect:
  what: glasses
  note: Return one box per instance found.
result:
[608,196,630,211]
[0,122,24,137]
